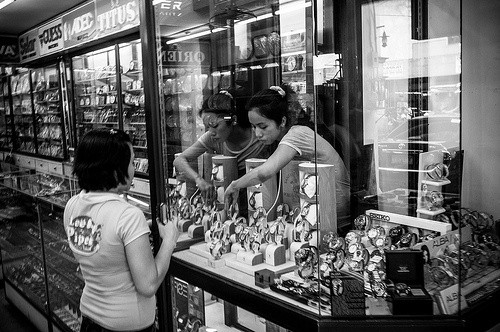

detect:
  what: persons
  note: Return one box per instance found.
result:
[224,83,350,234]
[63,127,180,332]
[172,89,273,219]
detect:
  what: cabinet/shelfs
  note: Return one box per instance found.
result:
[0,0,500,332]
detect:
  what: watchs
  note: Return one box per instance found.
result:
[175,162,500,306]
